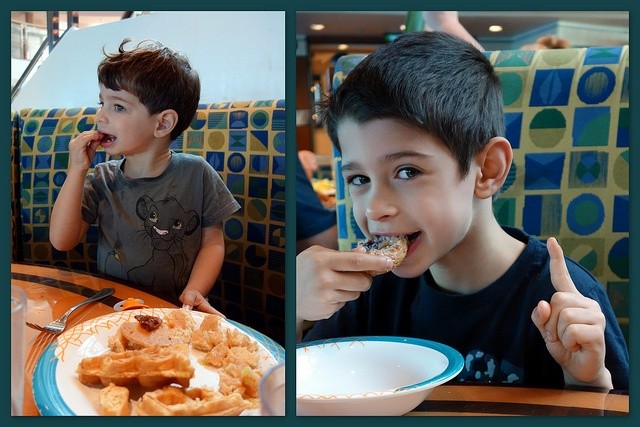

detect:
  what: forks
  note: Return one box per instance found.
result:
[26,287,115,334]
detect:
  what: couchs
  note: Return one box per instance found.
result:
[329,44,630,354]
[12,98,286,346]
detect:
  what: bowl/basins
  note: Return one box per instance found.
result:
[296,333,464,417]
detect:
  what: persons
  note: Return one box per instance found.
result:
[296,148,338,250]
[297,30,631,397]
[422,10,569,53]
[49,38,242,320]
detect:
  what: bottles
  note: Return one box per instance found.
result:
[11,280,26,414]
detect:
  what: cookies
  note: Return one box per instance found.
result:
[352,235,407,273]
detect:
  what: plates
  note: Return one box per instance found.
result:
[33,308,287,416]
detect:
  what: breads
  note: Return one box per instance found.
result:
[75,303,266,416]
